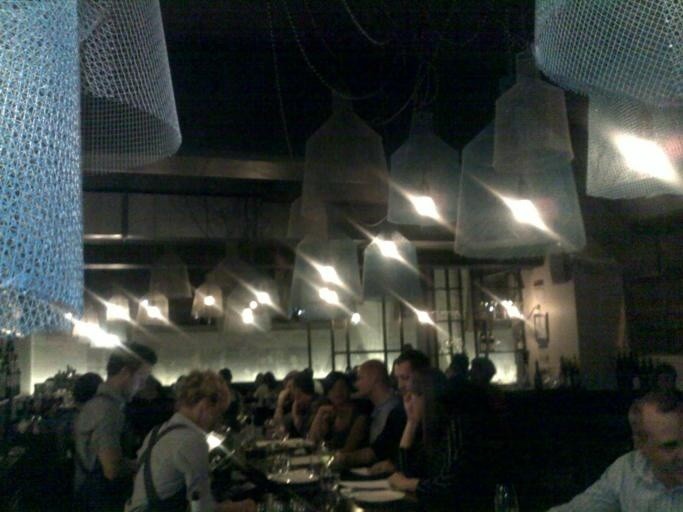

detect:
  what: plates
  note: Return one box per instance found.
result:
[235,416,407,504]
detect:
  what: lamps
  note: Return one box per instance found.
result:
[0,0,683,347]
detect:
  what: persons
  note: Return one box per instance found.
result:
[214,350,495,509]
[1,344,256,510]
[550,384,682,510]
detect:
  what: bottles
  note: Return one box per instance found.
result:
[533,346,666,395]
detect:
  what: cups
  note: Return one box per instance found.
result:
[493,480,519,512]
